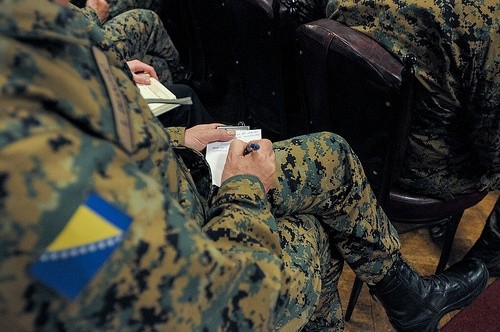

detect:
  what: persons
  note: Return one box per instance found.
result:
[0,0,500,332]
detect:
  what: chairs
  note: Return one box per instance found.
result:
[150,0,489,321]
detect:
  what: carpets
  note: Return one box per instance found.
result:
[437,278,500,332]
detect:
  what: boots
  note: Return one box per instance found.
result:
[368,252,490,332]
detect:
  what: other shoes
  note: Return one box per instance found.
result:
[431,225,452,242]
[179,71,216,92]
[463,240,500,277]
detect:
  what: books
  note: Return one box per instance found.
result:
[205,126,262,187]
[132,72,181,117]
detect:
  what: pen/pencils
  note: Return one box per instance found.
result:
[243,142,260,155]
